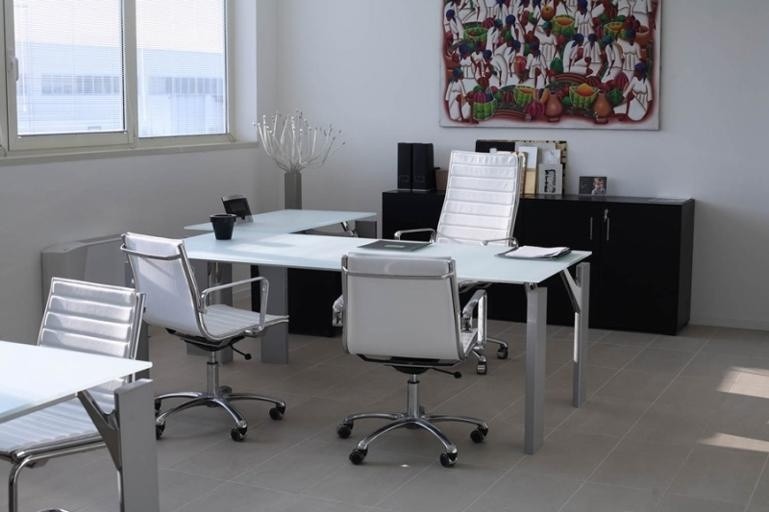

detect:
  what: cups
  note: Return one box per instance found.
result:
[210,212,236,240]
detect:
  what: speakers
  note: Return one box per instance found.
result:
[397,143,412,189]
[413,143,436,190]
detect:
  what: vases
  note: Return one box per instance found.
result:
[282,166,305,214]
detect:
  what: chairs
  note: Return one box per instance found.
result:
[394,147,524,360]
[6,278,146,512]
[122,231,288,441]
[333,249,488,468]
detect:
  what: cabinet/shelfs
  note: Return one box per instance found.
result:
[383,191,695,334]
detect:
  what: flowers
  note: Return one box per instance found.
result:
[250,111,344,171]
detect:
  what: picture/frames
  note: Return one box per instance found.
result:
[578,174,608,195]
[536,164,564,195]
[435,1,662,129]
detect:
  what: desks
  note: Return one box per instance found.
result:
[3,328,159,503]
[178,232,592,456]
[184,207,377,238]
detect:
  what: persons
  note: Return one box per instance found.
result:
[591,177,606,195]
